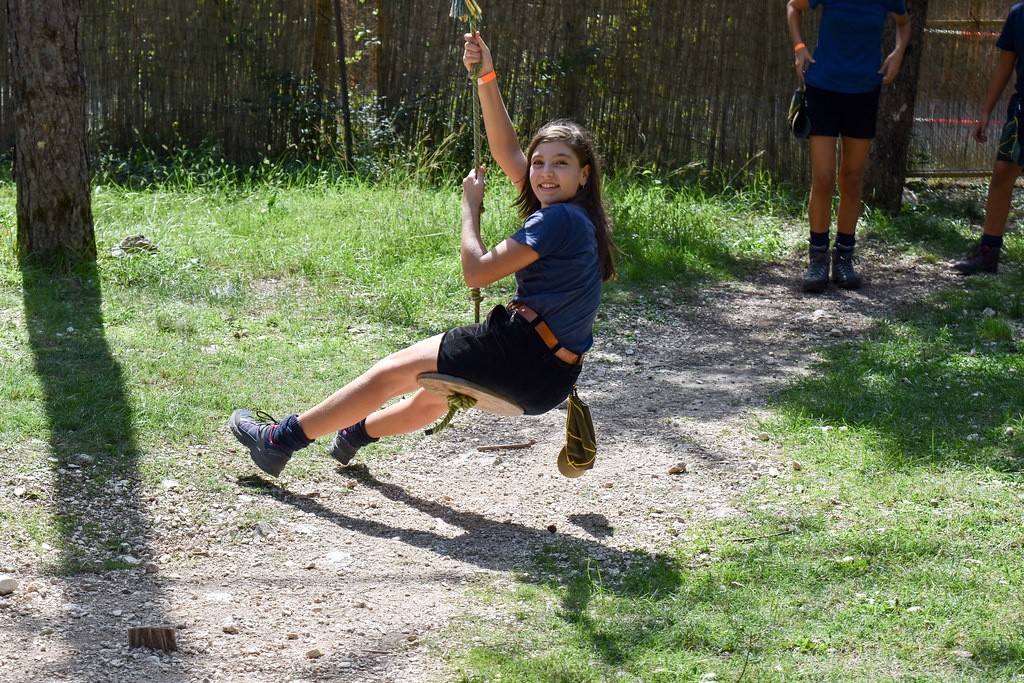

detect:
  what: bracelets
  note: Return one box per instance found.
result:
[794,42,806,53]
[475,69,497,86]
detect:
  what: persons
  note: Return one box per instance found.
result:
[229,30,618,477]
[787,0,913,290]
[954,0,1024,274]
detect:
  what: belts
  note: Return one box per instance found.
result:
[505,302,584,364]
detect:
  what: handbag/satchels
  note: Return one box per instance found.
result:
[787,83,811,139]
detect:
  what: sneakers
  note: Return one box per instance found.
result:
[954,244,1000,273]
[832,243,861,290]
[229,409,293,478]
[330,430,361,465]
[804,243,830,290]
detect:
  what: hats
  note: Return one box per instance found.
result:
[999,116,1020,164]
[557,387,597,478]
